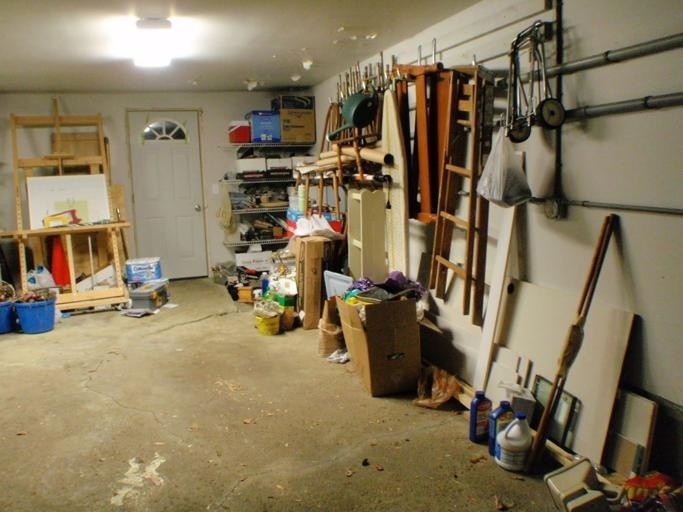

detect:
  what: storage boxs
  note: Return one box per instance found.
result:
[296,236,332,330]
[227,95,316,144]
[236,156,315,179]
[335,295,444,397]
[125,256,171,310]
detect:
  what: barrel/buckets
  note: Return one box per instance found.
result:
[495,412,532,471]
[0,301,14,335]
[14,297,56,334]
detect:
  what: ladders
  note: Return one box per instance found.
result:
[429,65,495,326]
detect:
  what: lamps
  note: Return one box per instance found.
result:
[132,19,172,67]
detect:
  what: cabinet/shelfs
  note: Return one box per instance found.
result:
[217,142,314,248]
[347,188,386,283]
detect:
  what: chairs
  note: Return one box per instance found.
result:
[333,92,384,183]
[295,100,342,222]
